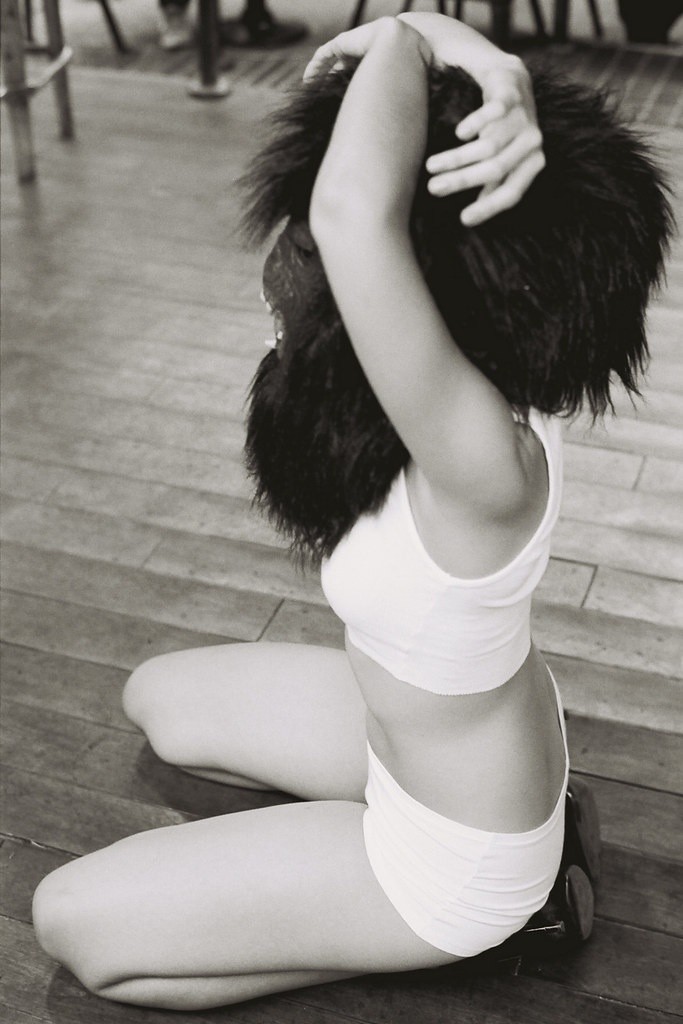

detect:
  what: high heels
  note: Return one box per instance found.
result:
[473,867,595,973]
[562,711,604,882]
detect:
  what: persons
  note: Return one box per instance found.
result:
[151,0,195,51]
[32,13,676,1011]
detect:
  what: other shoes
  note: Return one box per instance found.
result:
[162,21,193,50]
